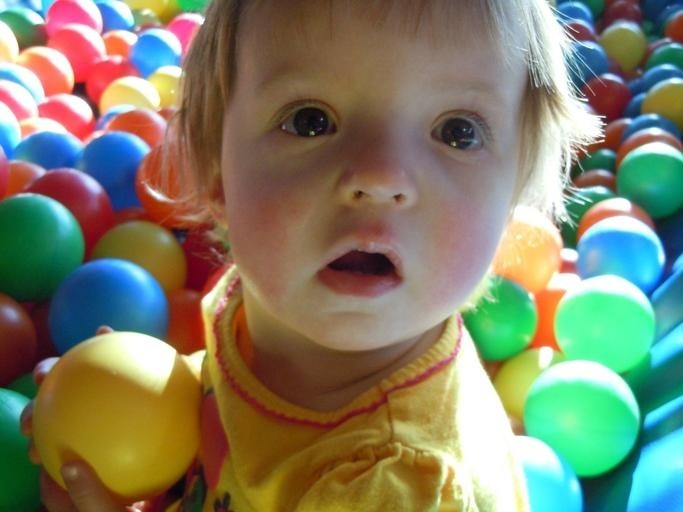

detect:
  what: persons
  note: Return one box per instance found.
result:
[19,0,606,511]
